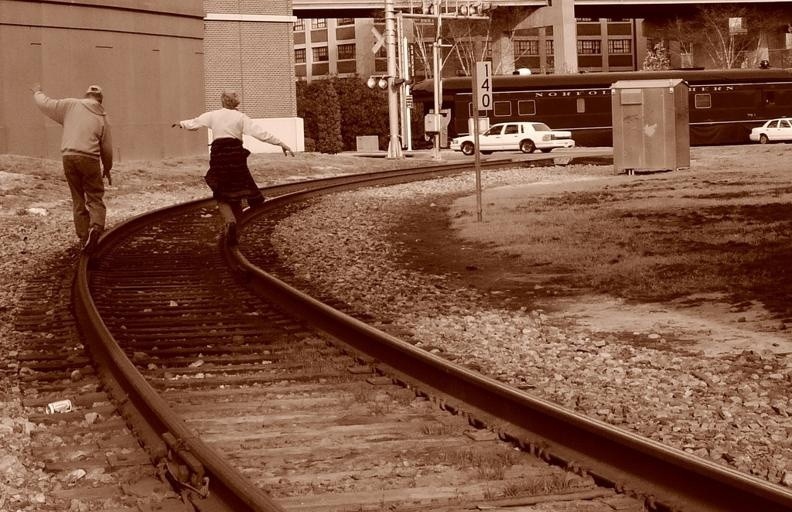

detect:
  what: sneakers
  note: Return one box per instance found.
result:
[225,221,241,247]
[80,228,98,255]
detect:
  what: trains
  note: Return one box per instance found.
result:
[409,61,792,146]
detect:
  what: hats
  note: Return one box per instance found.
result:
[86,85,103,103]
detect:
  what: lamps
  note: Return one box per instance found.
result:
[367,73,392,90]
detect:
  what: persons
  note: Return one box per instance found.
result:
[27,80,115,255]
[171,88,294,246]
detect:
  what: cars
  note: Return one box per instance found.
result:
[749,118,792,144]
[449,122,575,156]
